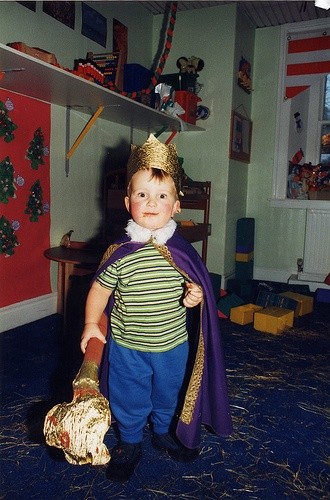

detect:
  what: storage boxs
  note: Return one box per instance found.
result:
[219,218,314,336]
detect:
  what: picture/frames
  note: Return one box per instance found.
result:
[229,109,253,163]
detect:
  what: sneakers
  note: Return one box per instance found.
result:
[105,440,144,483]
[153,433,198,459]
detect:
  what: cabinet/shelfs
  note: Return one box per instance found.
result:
[176,177,212,269]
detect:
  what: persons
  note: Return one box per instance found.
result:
[43,133,232,484]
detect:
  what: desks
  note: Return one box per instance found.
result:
[43,243,104,336]
